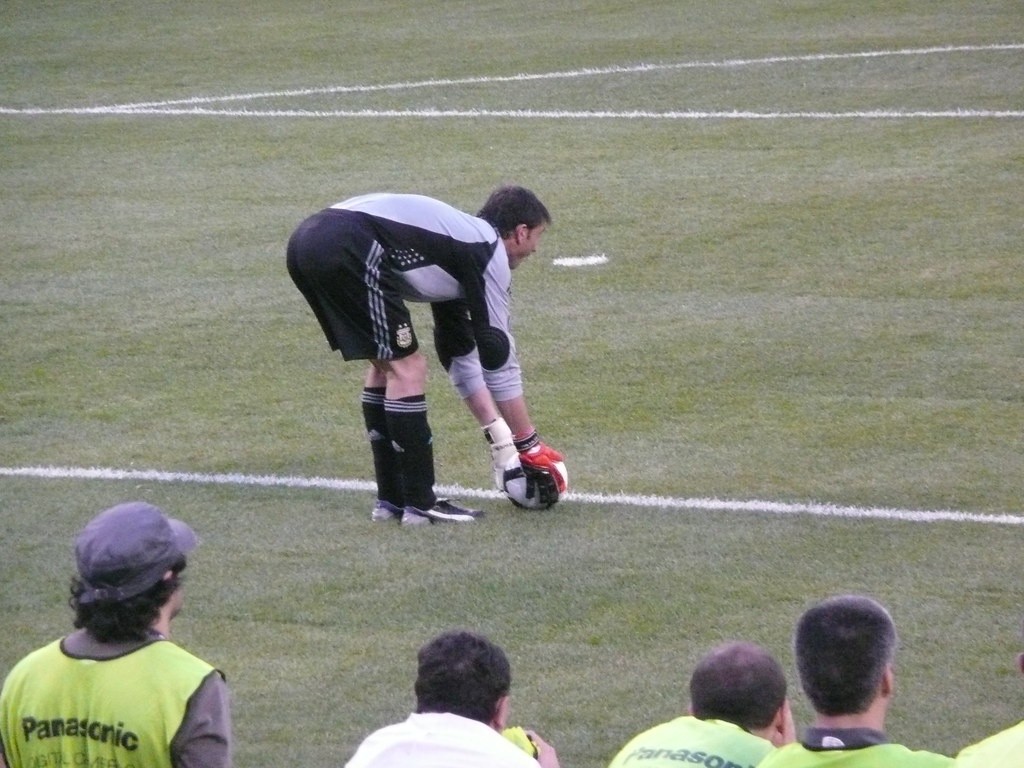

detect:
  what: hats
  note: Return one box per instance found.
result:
[69,500,199,602]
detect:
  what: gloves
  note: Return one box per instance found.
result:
[479,417,522,492]
[510,424,568,508]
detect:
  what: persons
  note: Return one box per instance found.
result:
[342,628,561,768]
[607,642,797,768]
[1,501,232,768]
[956,651,1024,768]
[750,594,958,768]
[286,186,569,526]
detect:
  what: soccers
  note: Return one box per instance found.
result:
[504,449,568,511]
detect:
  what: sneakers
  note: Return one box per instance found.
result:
[371,499,403,522]
[401,496,484,528]
